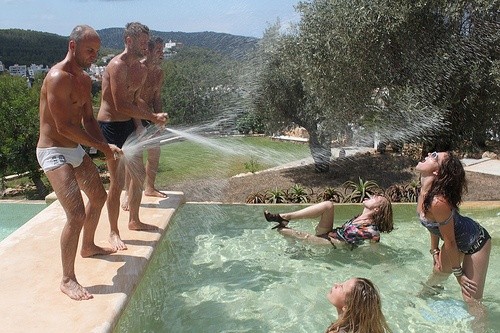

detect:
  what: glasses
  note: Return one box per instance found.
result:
[431,151,440,165]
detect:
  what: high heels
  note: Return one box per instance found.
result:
[264,212,289,229]
